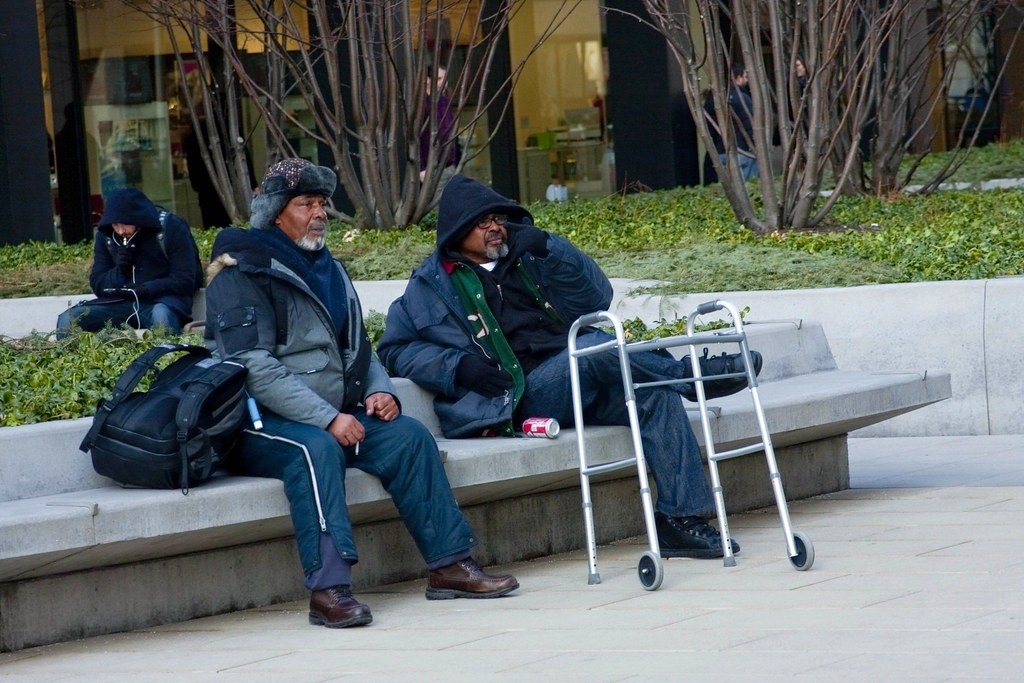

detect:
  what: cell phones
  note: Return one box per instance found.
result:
[103,287,131,294]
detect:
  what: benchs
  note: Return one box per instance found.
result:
[0,319,954,656]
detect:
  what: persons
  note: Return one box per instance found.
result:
[405,66,462,209]
[702,58,812,184]
[54,102,97,242]
[55,185,201,339]
[183,102,259,229]
[203,159,521,628]
[376,175,762,559]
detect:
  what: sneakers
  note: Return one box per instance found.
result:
[654,512,740,558]
[681,348,762,402]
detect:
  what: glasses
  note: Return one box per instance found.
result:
[476,214,507,229]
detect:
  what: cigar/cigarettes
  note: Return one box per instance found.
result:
[355,441,359,456]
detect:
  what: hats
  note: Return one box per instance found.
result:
[250,158,336,231]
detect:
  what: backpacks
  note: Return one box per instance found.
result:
[79,344,249,495]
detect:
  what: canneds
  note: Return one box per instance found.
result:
[522,417,560,438]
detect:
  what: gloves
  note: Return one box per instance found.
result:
[117,246,132,274]
[121,284,144,303]
[454,354,513,396]
[503,217,550,263]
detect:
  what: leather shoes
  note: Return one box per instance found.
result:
[309,588,372,628]
[425,556,519,601]
